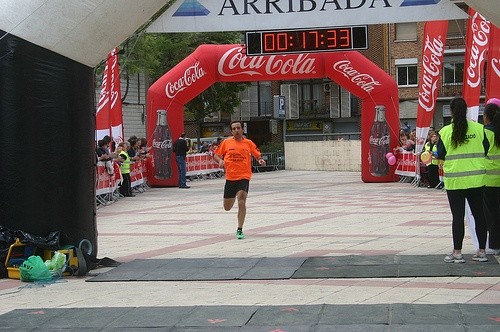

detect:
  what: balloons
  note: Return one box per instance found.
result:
[484,97,500,107]
[420,152,432,164]
[386,152,393,159]
[388,155,396,166]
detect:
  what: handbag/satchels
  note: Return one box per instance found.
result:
[19,255,53,282]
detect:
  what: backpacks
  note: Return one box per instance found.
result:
[173,141,183,152]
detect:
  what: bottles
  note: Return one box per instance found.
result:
[369,106,390,176]
[152,109,172,179]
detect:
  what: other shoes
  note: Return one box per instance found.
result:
[127,194,135,197]
[427,186,430,187]
[179,185,190,188]
[439,186,442,188]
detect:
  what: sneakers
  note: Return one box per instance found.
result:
[472,252,488,261]
[236,228,244,239]
[493,249,500,256]
[444,253,466,263]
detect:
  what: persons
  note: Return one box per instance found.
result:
[199,137,224,178]
[214,120,266,239]
[393,127,440,189]
[438,97,490,263]
[172,132,191,189]
[484,103,500,256]
[96,135,153,197]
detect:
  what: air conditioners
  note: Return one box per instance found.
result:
[324,84,332,93]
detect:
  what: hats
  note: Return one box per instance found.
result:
[181,132,186,135]
